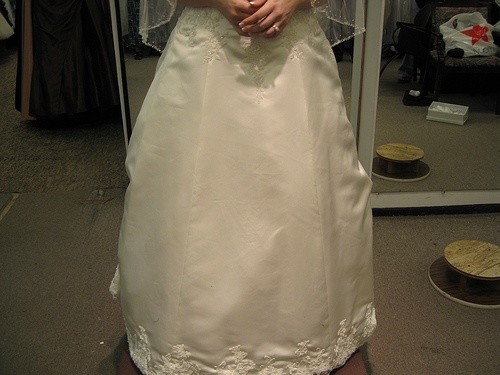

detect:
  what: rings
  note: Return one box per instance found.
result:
[272,25,280,33]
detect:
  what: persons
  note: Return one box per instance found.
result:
[13,0,119,127]
[109,0,377,375]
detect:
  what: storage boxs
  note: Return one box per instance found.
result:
[425,101,469,126]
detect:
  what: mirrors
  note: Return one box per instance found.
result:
[111,0,356,147]
[358,0,500,211]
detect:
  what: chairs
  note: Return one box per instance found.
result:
[379,3,433,88]
[419,0,500,115]
